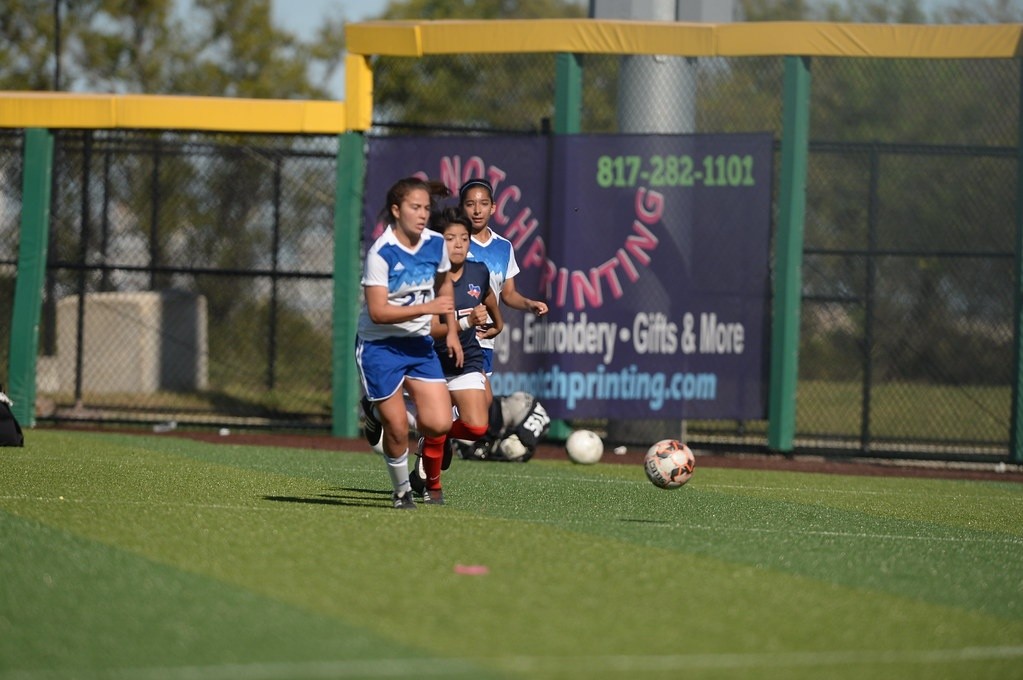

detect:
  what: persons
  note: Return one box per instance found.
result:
[353,177,463,510]
[373,178,570,504]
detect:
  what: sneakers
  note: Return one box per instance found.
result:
[441,437,452,470]
[392,488,417,509]
[415,437,427,481]
[421,487,445,506]
[372,427,384,456]
[360,395,385,446]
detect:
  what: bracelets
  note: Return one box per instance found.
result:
[459,317,470,331]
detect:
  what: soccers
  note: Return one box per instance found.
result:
[642,437,696,489]
[565,429,605,466]
